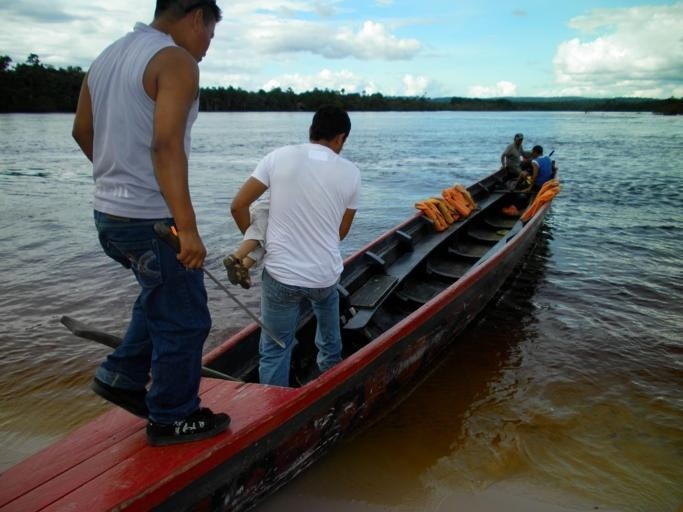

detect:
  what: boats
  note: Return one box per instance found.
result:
[0,155,560,511]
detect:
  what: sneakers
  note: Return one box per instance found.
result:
[90,376,150,419]
[147,407,232,447]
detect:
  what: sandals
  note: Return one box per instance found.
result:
[223,254,251,289]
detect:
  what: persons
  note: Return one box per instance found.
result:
[225,199,269,290]
[68,0,231,447]
[232,105,360,386]
[501,133,528,178]
[511,146,553,195]
